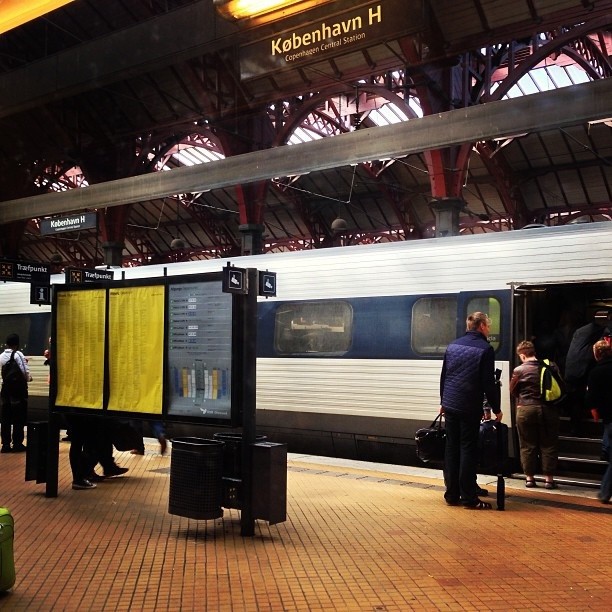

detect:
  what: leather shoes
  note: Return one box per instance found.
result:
[159,434,167,454]
[131,450,144,455]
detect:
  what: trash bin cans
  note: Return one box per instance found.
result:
[213,432,268,509]
[168,436,226,520]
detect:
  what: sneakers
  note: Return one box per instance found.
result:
[62,436,70,441]
[104,465,128,476]
[1,444,12,453]
[87,470,105,481]
[597,492,609,502]
[73,479,97,489]
[476,484,488,496]
[12,444,26,453]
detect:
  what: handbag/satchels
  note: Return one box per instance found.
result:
[539,358,567,408]
[414,414,447,463]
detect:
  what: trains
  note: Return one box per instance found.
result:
[1,219,612,498]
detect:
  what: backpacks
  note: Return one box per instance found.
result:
[2,350,28,401]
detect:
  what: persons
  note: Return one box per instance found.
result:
[1,333,33,452]
[56,413,97,490]
[89,415,130,480]
[508,340,559,488]
[440,311,503,510]
[562,310,607,411]
[588,340,612,502]
[44,337,72,441]
[131,415,167,456]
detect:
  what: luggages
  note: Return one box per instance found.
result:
[0,507,15,594]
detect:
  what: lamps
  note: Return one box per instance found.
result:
[215,0,304,20]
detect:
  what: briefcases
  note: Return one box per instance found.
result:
[480,420,508,476]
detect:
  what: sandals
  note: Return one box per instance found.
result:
[464,498,491,509]
[544,482,558,488]
[444,496,463,506]
[526,479,536,486]
